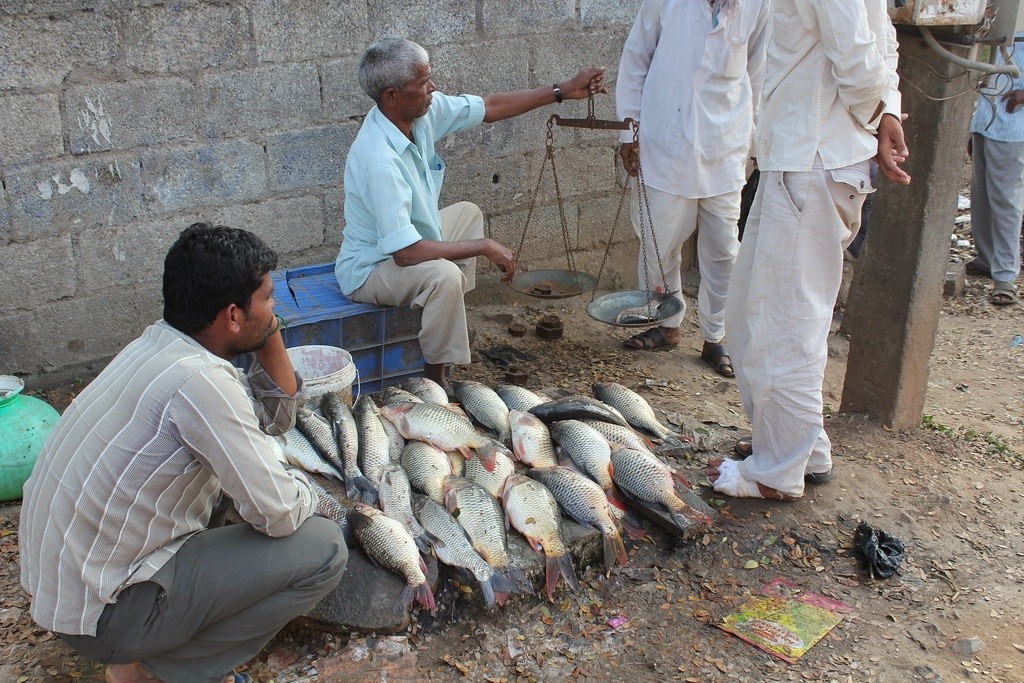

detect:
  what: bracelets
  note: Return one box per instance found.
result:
[269,314,289,336]
[553,84,562,103]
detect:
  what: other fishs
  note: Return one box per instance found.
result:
[615,289,678,324]
[278,377,714,590]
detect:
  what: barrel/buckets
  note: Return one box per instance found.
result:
[285,345,361,419]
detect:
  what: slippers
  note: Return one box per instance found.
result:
[736,432,836,483]
[965,260,991,276]
[625,328,679,352]
[219,674,245,683]
[991,280,1019,304]
[701,346,735,378]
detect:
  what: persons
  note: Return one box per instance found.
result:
[616,0,770,378]
[706,0,911,499]
[18,224,349,683]
[335,37,608,407]
[965,32,1024,304]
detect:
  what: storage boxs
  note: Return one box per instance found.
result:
[228,261,429,399]
